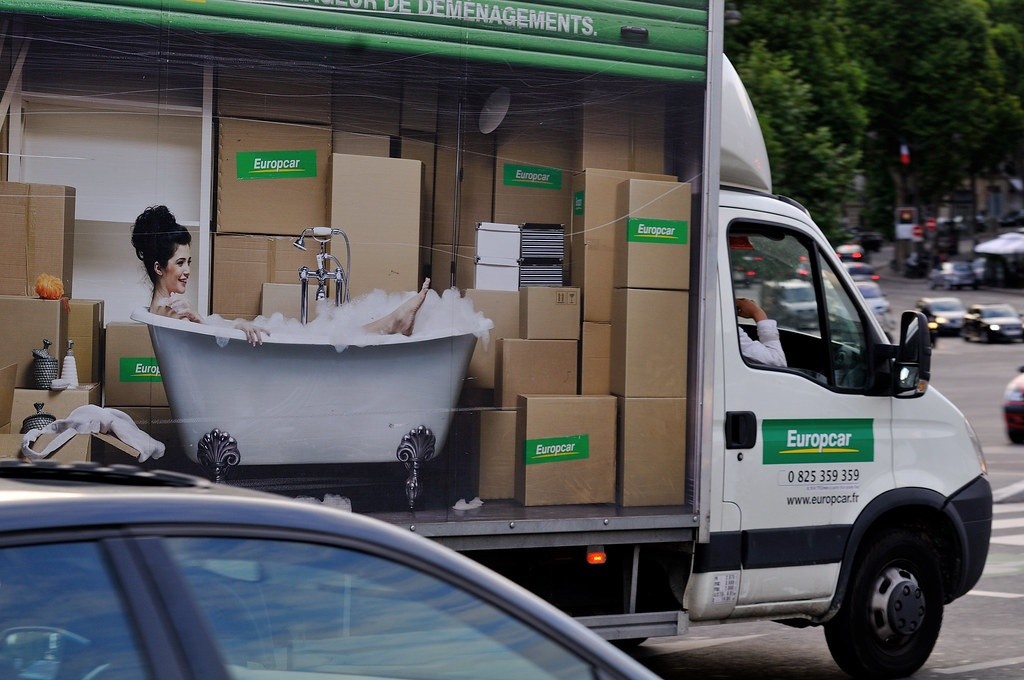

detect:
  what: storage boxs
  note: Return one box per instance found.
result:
[0,38,690,507]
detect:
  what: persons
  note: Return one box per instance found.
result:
[735,298,788,369]
[130,205,431,347]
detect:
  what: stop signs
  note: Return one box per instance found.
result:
[913,226,922,237]
[925,218,937,231]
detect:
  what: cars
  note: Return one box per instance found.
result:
[1003,366,1024,444]
[928,257,1002,291]
[959,303,1024,343]
[0,460,666,680]
[730,243,892,334]
[915,298,967,333]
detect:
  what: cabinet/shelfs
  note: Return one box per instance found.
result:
[0,37,214,320]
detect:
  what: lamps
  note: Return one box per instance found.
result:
[449,71,513,289]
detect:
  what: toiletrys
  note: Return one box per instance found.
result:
[59,339,79,389]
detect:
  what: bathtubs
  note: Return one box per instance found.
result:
[129,307,494,465]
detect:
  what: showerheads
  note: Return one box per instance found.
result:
[455,76,511,135]
[292,227,350,252]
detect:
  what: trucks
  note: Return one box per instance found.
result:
[0,0,995,680]
